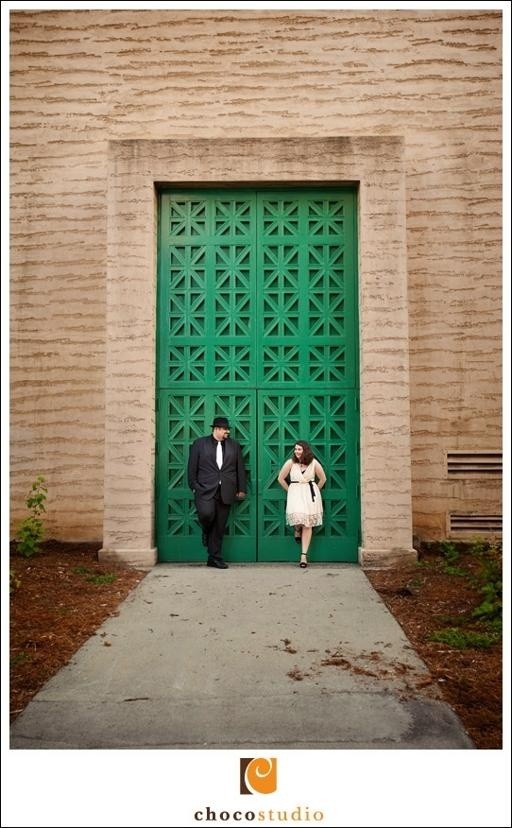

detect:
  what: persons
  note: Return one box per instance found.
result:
[188,417,246,569]
[277,440,327,568]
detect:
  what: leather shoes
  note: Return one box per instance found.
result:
[207,557,228,568]
[202,531,208,546]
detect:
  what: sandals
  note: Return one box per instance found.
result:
[300,553,307,568]
[294,528,301,543]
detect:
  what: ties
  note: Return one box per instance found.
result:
[215,441,223,485]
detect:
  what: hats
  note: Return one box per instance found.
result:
[210,418,232,428]
